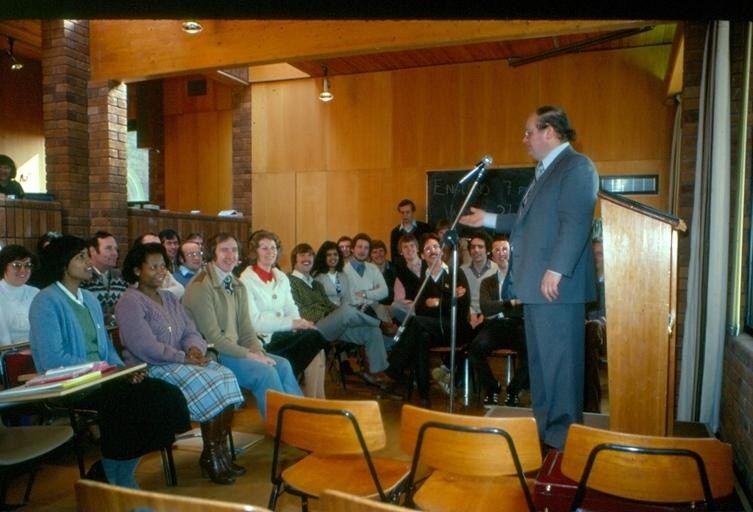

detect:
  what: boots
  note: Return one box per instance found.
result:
[220,405,247,477]
[199,416,235,484]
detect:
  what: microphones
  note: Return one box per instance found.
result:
[456,155,495,185]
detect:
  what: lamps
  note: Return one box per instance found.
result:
[318,65,333,101]
[5,37,22,70]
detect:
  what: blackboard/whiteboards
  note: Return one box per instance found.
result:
[426,165,537,239]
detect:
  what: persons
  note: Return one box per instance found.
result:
[0,155,24,200]
[459,105,600,463]
[0,200,528,484]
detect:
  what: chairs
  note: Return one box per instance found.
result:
[432,346,469,404]
[397,403,543,510]
[489,349,519,397]
[1,321,180,509]
[319,488,400,510]
[263,390,410,509]
[559,424,734,509]
[74,480,257,510]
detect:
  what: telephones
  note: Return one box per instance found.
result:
[217,209,242,218]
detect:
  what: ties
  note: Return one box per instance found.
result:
[524,162,544,206]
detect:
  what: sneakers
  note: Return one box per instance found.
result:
[482,390,501,409]
[440,382,457,398]
[433,368,451,383]
[504,391,520,408]
[364,370,392,388]
[381,322,398,336]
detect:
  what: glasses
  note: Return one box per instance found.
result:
[12,261,34,271]
